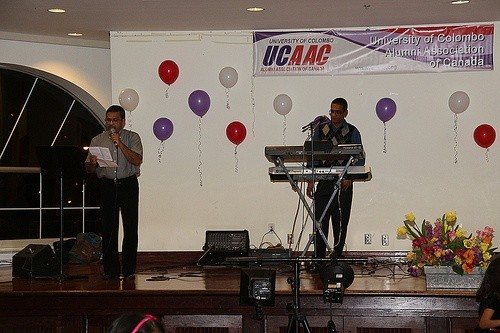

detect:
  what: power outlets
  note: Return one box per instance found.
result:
[365,234,372,244]
[309,234,315,244]
[268,223,275,234]
[382,235,389,246]
[287,233,294,244]
[368,257,374,264]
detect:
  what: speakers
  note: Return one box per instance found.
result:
[12,244,59,279]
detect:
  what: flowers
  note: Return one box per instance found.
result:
[395,208,498,278]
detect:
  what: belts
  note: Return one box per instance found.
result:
[102,177,133,185]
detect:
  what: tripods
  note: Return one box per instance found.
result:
[34,144,89,284]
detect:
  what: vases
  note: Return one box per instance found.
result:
[423,266,487,288]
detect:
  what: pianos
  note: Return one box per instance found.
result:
[264,143,373,259]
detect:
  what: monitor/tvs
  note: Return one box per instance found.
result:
[239,269,276,306]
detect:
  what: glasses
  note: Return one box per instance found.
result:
[329,109,346,115]
[105,116,122,122]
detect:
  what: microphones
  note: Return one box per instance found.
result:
[110,128,118,148]
[319,115,327,124]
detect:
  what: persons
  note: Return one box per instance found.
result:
[473,258,500,333]
[111,312,166,333]
[306,98,365,259]
[84,105,143,279]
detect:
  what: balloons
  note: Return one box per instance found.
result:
[272,93,293,115]
[188,89,210,117]
[219,67,238,89]
[473,124,496,151]
[449,90,470,115]
[153,117,174,142]
[158,60,180,86]
[375,98,397,123]
[119,88,140,112]
[225,121,247,145]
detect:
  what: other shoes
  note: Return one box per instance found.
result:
[102,274,120,280]
[123,273,136,279]
